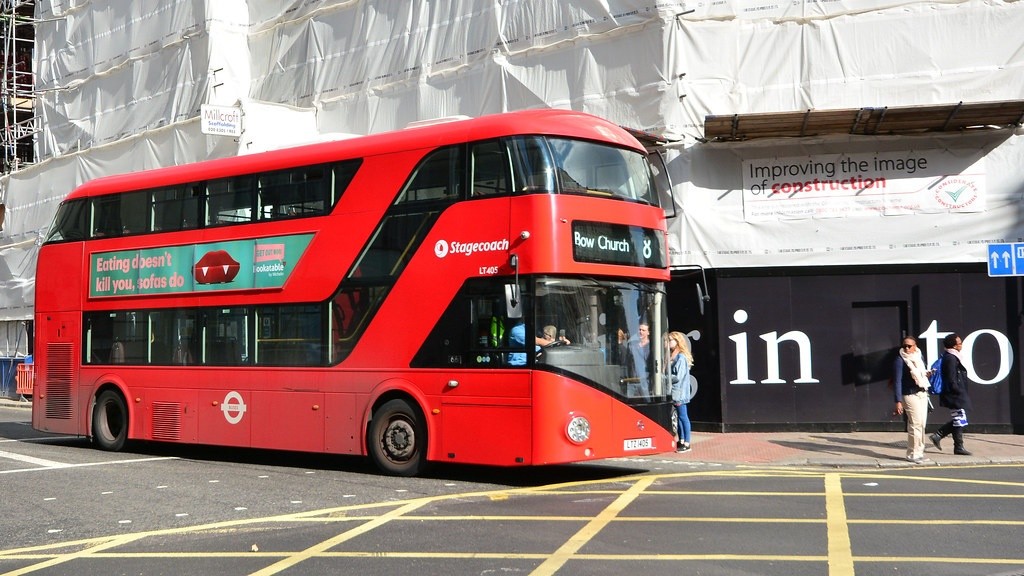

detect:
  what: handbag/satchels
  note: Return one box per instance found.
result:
[928,397,934,414]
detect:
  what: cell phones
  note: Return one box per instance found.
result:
[929,369,937,373]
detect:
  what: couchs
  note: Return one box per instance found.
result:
[108,336,303,366]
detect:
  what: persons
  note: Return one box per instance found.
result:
[661,333,668,375]
[544,325,557,343]
[894,334,932,464]
[628,322,650,395]
[510,325,571,365]
[617,328,626,361]
[929,334,974,457]
[669,331,693,452]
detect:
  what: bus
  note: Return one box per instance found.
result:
[33,109,712,478]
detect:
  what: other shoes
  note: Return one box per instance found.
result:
[912,457,930,463]
[677,441,684,448]
[678,444,691,453]
[954,446,972,455]
[929,434,942,451]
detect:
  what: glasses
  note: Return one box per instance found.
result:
[903,344,914,349]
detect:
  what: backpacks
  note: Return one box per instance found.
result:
[929,353,944,394]
